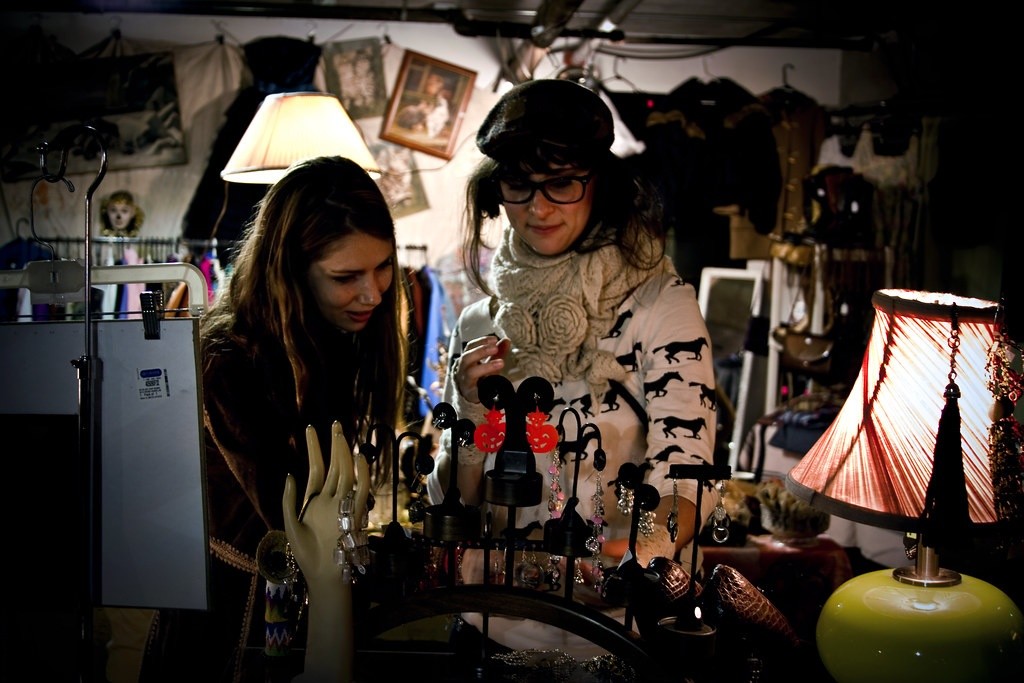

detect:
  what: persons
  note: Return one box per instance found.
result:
[134,157,452,683]
[101,187,144,266]
[424,79,722,683]
[282,421,370,683]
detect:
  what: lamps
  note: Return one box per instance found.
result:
[221,91,383,186]
[786,290,1024,683]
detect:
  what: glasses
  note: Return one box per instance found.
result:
[498,170,595,204]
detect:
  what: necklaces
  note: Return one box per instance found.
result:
[335,489,370,585]
[284,489,319,634]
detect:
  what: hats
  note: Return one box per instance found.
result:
[476,79,615,161]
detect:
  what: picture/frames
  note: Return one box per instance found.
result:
[325,36,391,118]
[379,49,478,159]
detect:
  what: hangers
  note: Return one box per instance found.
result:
[770,64,800,98]
[691,50,723,106]
[15,219,220,266]
